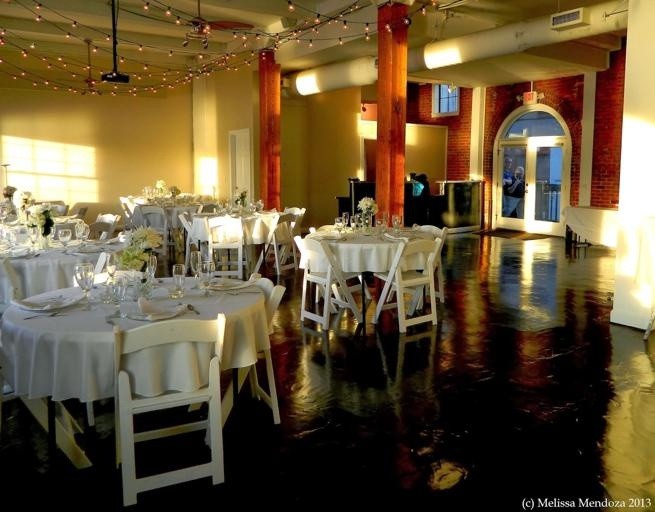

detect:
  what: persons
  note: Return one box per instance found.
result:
[502,156,522,218]
[503,166,525,219]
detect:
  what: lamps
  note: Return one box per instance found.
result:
[365,33,370,40]
[315,13,321,24]
[365,23,368,30]
[66,33,70,37]
[106,35,110,40]
[22,49,26,53]
[166,6,171,15]
[287,0,295,12]
[343,20,348,30]
[93,47,97,52]
[139,45,142,51]
[36,16,41,20]
[30,42,36,49]
[169,50,172,56]
[144,2,149,10]
[23,52,27,56]
[72,21,76,27]
[121,57,124,62]
[2,29,6,35]
[339,37,343,45]
[309,39,312,47]
[144,64,147,70]
[36,4,41,9]
[175,16,180,24]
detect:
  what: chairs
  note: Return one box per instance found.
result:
[253,213,298,275]
[178,211,208,259]
[371,237,442,332]
[139,204,176,256]
[77,206,88,220]
[387,223,449,316]
[86,222,111,241]
[204,215,246,280]
[294,235,363,331]
[95,213,121,240]
[52,204,69,216]
[265,207,306,268]
[111,311,226,506]
[0,368,92,469]
[185,204,218,266]
[309,226,371,309]
[184,271,286,443]
[117,202,133,230]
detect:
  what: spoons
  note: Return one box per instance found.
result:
[187,304,200,315]
[24,312,61,320]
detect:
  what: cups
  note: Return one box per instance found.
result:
[335,217,345,239]
[146,255,159,283]
[106,255,119,288]
[58,229,72,253]
[173,263,186,298]
[392,214,398,232]
[28,227,40,255]
[0,208,8,226]
[105,276,128,321]
[375,219,386,240]
[342,212,349,234]
[73,263,102,304]
[196,260,217,297]
[350,216,358,235]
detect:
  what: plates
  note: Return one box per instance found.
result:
[0,252,29,260]
[384,232,418,243]
[198,281,250,290]
[20,298,73,310]
[126,306,180,321]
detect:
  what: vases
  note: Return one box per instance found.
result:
[361,212,372,235]
[133,254,157,299]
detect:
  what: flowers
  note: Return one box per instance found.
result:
[120,225,163,283]
[35,205,54,237]
[358,197,379,236]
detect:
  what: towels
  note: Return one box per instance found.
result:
[381,232,411,243]
[193,272,262,290]
[130,297,187,320]
[9,290,89,314]
